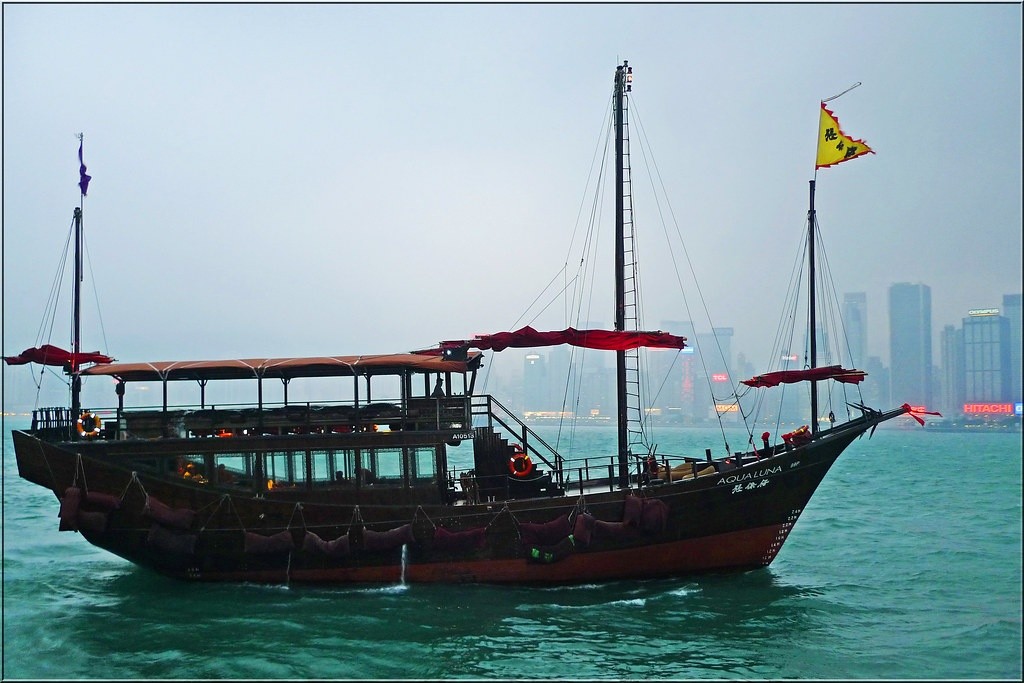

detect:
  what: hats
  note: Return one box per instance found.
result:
[436,378,443,381]
[219,464,225,468]
[336,471,343,475]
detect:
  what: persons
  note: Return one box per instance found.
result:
[354,467,377,484]
[335,470,345,481]
[433,377,446,397]
[184,465,196,480]
[217,464,229,482]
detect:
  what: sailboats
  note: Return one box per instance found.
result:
[0,54,940,588]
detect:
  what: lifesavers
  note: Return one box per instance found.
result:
[792,425,809,436]
[509,443,522,451]
[77,413,102,437]
[509,454,533,475]
